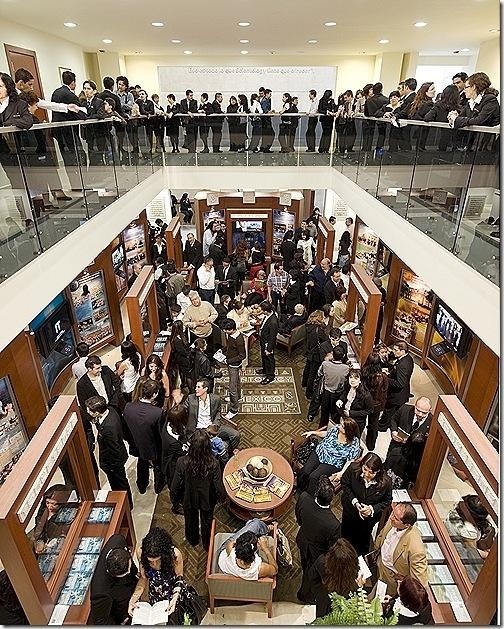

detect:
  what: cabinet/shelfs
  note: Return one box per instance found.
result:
[47,490,137,626]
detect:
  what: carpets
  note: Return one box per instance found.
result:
[145,340,343,605]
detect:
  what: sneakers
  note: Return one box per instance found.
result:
[225,397,242,402]
[225,411,238,419]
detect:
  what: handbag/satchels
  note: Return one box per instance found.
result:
[255,108,259,113]
[297,438,319,465]
[172,576,208,625]
[277,528,293,568]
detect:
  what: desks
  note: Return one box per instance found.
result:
[223,447,295,520]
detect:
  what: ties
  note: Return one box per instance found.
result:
[412,420,419,430]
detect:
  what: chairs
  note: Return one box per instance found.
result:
[291,434,369,500]
[205,520,277,618]
[276,322,306,357]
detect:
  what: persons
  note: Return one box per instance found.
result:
[1,70,500,279]
[32,483,72,540]
[462,493,495,547]
[72,196,434,626]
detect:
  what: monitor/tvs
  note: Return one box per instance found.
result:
[34,298,75,359]
[429,298,470,360]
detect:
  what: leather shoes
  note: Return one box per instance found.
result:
[56,197,72,201]
[171,505,185,515]
[44,204,59,210]
[172,149,180,153]
[256,369,266,374]
[306,149,357,153]
[182,145,223,153]
[279,146,295,153]
[260,376,274,385]
[228,147,274,153]
[308,414,314,422]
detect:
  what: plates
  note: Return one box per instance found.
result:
[244,456,273,480]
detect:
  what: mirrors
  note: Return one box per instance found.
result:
[424,438,499,596]
[24,439,89,597]
[140,302,152,349]
[350,290,368,350]
